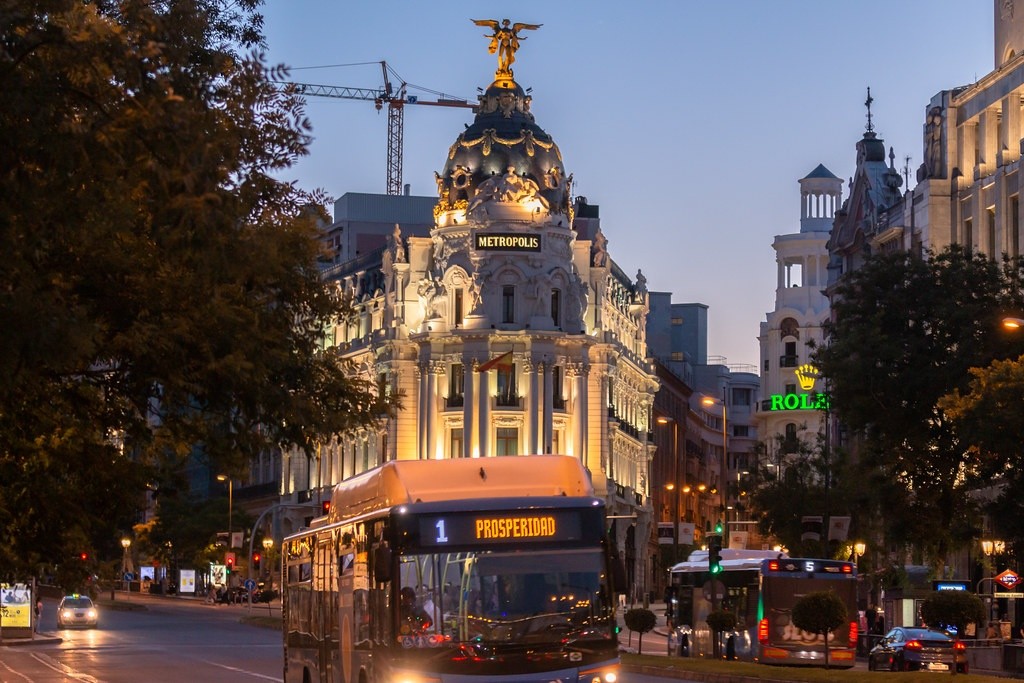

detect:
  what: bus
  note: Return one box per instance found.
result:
[669,545,857,668]
[962,593,1024,639]
[279,454,622,679]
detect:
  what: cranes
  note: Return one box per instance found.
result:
[253,60,479,197]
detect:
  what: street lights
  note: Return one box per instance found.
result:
[654,414,680,555]
[701,394,728,549]
[120,533,131,590]
[217,475,234,552]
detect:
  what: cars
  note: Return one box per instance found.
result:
[57,594,99,629]
[869,627,969,675]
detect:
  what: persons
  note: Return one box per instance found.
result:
[619,591,626,612]
[35,597,44,635]
[636,269,646,282]
[470,19,544,71]
[593,228,608,266]
[532,275,553,317]
[1020,622,1024,639]
[865,603,884,635]
[499,165,518,193]
[220,583,227,595]
[986,622,995,639]
[472,275,488,314]
[400,583,440,631]
[392,223,402,244]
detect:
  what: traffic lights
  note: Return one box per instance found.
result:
[226,558,233,574]
[253,555,259,572]
[707,535,723,574]
